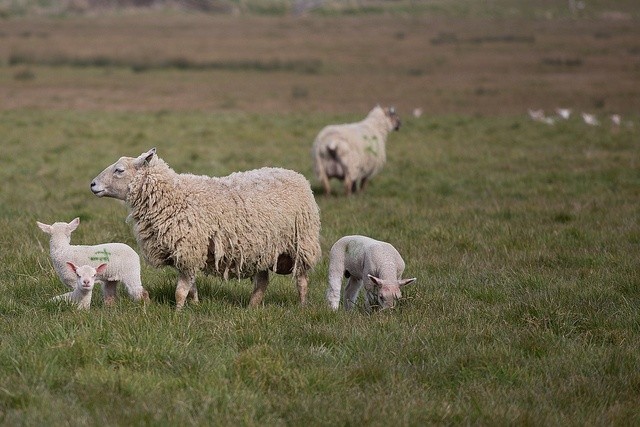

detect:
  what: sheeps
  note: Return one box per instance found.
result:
[90,144,325,318]
[312,103,403,196]
[44,261,107,313]
[323,234,421,319]
[31,212,153,309]
